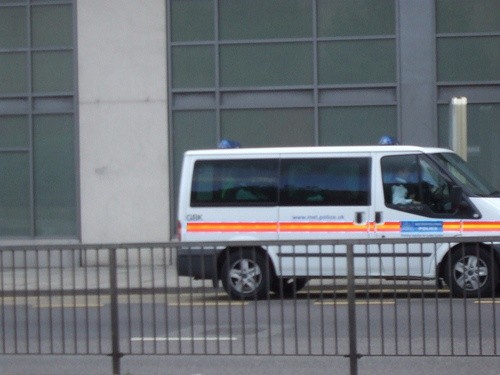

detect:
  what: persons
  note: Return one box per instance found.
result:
[391,158,423,206]
[234,164,258,201]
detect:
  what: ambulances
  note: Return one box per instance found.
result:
[174,136,499,300]
